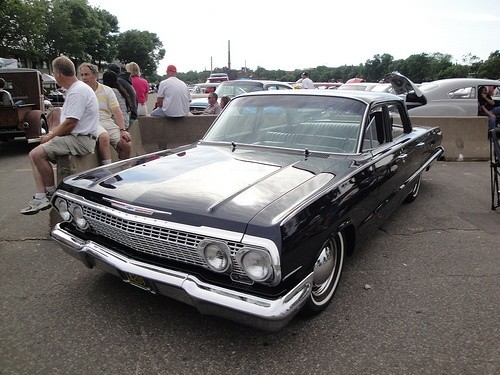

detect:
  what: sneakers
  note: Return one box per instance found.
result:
[20,196,52,215]
[32,192,55,202]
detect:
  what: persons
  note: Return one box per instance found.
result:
[152,82,160,93]
[478,87,495,115]
[0,78,24,105]
[106,64,138,126]
[202,92,222,114]
[151,65,192,117]
[220,96,231,109]
[20,55,99,216]
[77,61,132,166]
[299,72,314,89]
[101,69,131,129]
[123,62,148,117]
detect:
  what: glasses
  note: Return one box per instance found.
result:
[51,71,58,77]
[208,97,214,99]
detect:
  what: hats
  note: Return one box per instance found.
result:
[167,65,176,72]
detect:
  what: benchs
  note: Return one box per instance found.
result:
[264,131,381,152]
[292,123,404,138]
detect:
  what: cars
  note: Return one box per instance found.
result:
[0,58,500,154]
[49,90,445,332]
